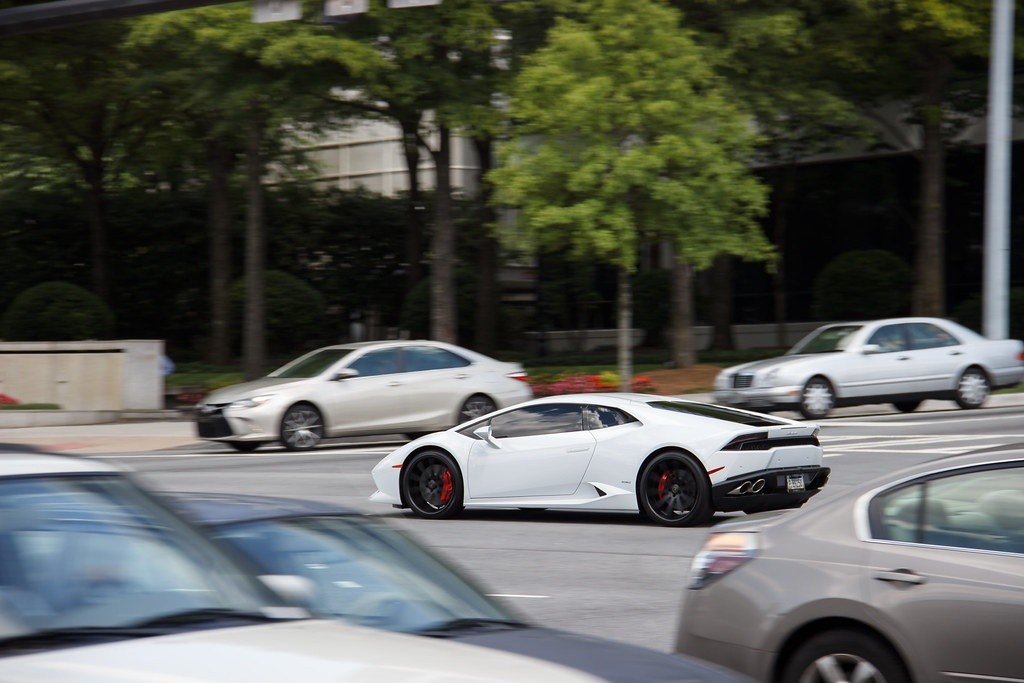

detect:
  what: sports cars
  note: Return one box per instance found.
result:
[368,390,832,526]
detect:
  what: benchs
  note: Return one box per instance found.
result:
[892,491,1024,553]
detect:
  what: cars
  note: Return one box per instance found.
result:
[192,339,536,454]
[0,450,603,683]
[673,440,1024,683]
[0,487,763,682]
[712,316,1024,420]
